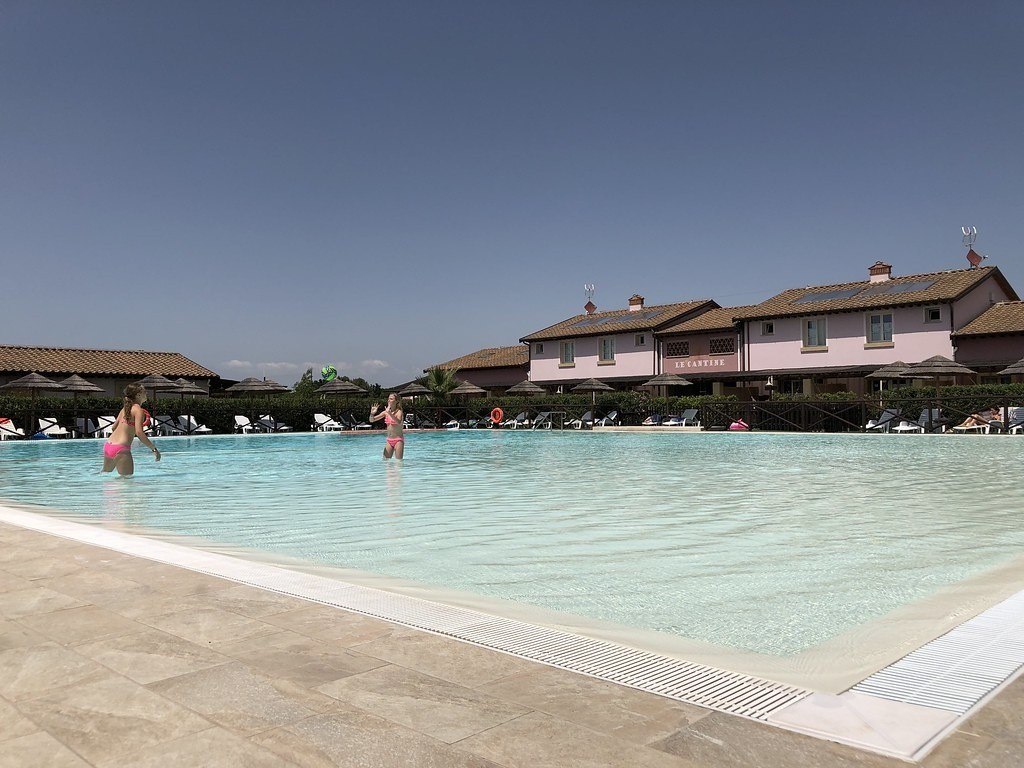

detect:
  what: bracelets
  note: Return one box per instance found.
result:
[152,448,157,452]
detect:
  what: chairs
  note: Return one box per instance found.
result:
[517,412,551,430]
[999,407,1024,434]
[314,414,343,431]
[893,410,943,433]
[351,415,365,425]
[595,411,618,427]
[260,415,292,433]
[0,415,212,441]
[499,412,528,429]
[642,415,678,426]
[954,407,1014,434]
[564,411,596,429]
[234,415,261,434]
[339,416,372,430]
[403,414,414,429]
[443,419,479,429]
[866,408,900,433]
[664,409,699,426]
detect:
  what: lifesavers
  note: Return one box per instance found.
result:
[490,407,504,423]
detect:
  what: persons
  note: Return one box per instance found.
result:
[99,382,161,477]
[369,392,405,461]
[957,405,1001,429]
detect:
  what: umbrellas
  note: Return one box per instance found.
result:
[505,380,546,421]
[398,382,434,423]
[446,380,487,426]
[570,377,615,419]
[44,373,106,425]
[134,372,368,429]
[0,372,69,434]
[641,371,694,411]
[899,354,979,423]
[996,357,1024,376]
[864,358,934,428]
